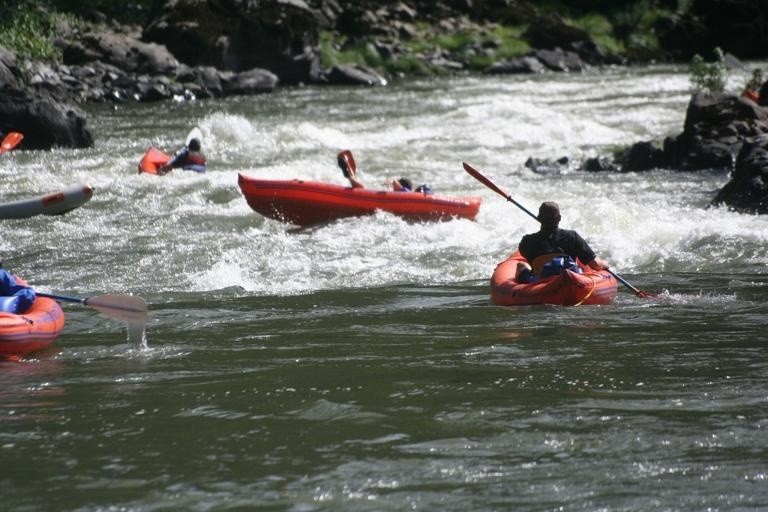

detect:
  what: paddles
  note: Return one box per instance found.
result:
[338,150,356,177]
[0,131,24,154]
[463,162,655,298]
[35,291,149,325]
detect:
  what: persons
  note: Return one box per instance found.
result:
[746,67,765,94]
[1,258,36,315]
[157,137,206,173]
[514,200,609,284]
[337,158,415,193]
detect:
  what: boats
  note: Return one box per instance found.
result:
[0,272,66,353]
[237,171,483,230]
[136,145,174,177]
[0,185,96,220]
[739,88,759,101]
[490,248,618,306]
